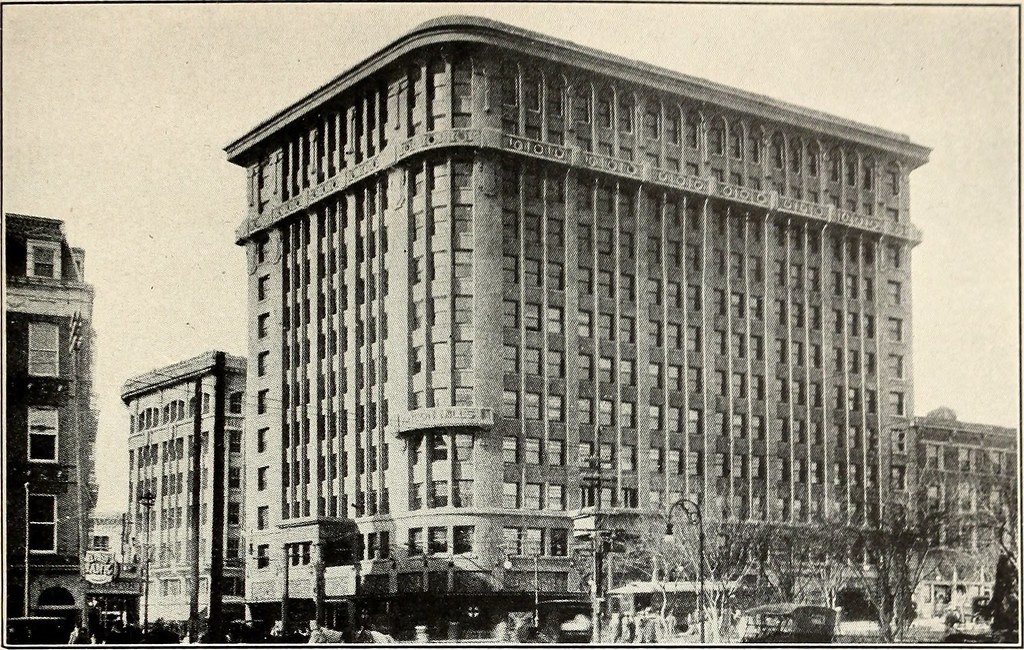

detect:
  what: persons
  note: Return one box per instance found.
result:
[270,620,284,636]
[90,634,96,644]
[179,631,190,645]
[339,620,377,644]
[68,624,84,644]
[834,607,843,638]
[687,607,741,637]
[496,612,537,644]
[940,608,961,639]
[308,620,327,643]
[609,606,678,643]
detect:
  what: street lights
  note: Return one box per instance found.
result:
[663,498,705,644]
[504,551,541,631]
[137,488,156,636]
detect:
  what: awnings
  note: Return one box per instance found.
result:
[607,580,748,595]
